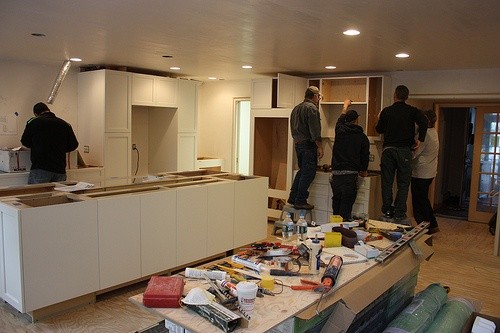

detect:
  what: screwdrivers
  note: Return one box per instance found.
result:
[269,269,314,276]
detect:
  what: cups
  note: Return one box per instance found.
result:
[261,275,274,290]
[236,282,258,310]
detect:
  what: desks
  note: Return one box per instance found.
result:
[128,219,429,333]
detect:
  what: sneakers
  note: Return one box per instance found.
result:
[392,211,407,220]
[383,210,394,218]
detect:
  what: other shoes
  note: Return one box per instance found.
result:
[333,213,353,222]
[287,198,314,210]
[425,226,440,235]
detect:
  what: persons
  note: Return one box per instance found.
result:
[375,85,428,221]
[411,110,439,235]
[330,99,370,221]
[286,86,324,210]
[20,102,79,185]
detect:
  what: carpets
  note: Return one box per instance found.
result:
[436,201,498,218]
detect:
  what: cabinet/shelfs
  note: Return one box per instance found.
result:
[248,73,392,224]
[78,68,198,189]
[0,170,269,320]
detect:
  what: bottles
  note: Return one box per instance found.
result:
[308,234,321,274]
[281,213,294,244]
[185,268,231,280]
[232,255,268,271]
[296,213,307,244]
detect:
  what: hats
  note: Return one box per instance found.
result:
[344,110,359,123]
